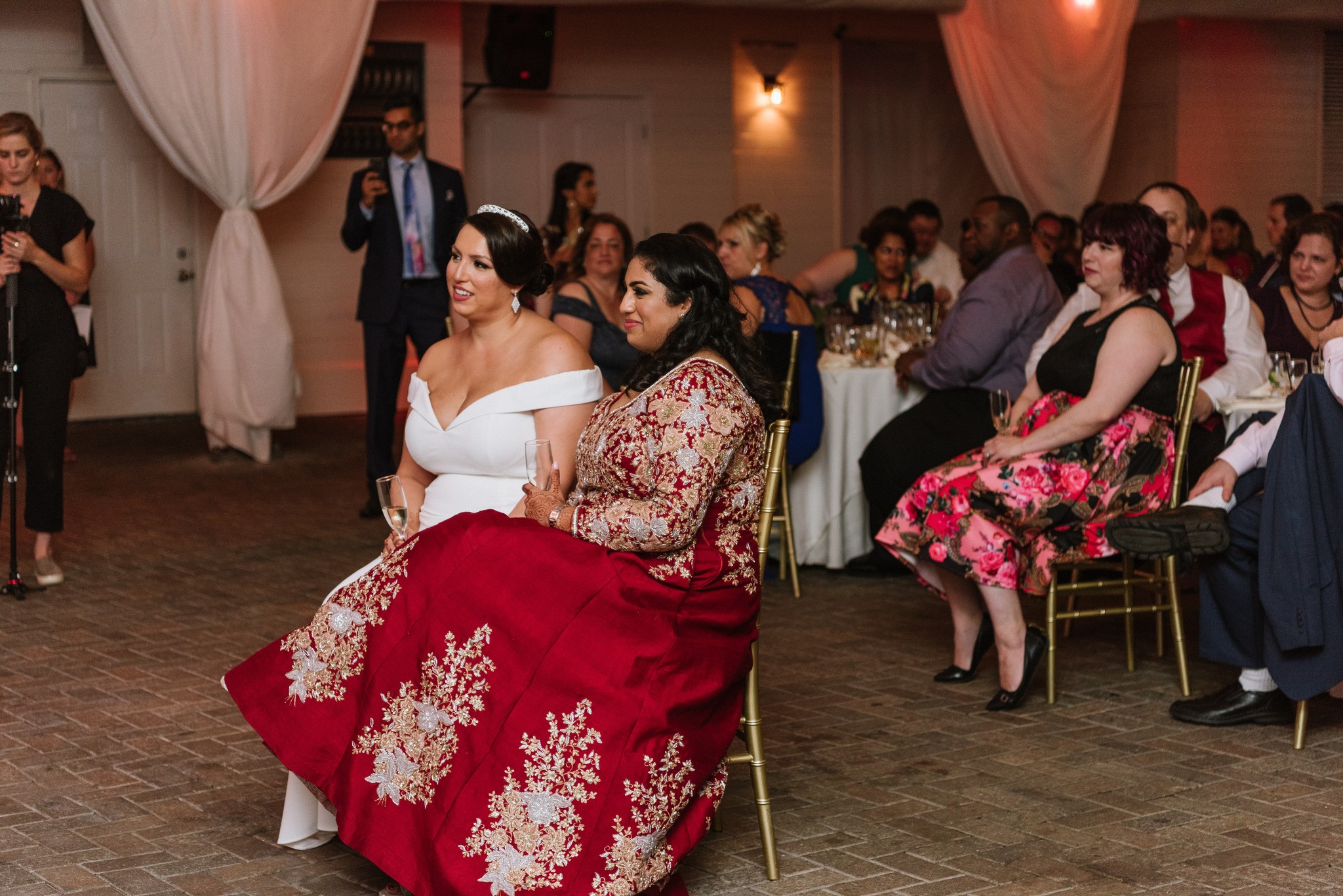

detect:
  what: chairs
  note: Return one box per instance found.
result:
[1007,355,1207,704]
[762,326,808,600]
[717,417,796,883]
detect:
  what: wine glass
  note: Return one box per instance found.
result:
[1264,351,1325,399]
[822,296,942,369]
[525,439,553,492]
[989,389,1010,436]
[377,475,408,541]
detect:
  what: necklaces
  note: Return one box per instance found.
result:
[541,224,584,254]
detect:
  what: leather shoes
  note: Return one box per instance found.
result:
[986,624,1049,712]
[1168,677,1294,729]
[1103,506,1233,561]
[934,613,994,683]
[358,500,381,518]
[846,550,910,577]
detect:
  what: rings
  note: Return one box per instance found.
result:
[985,451,990,457]
[523,512,525,516]
[381,551,389,559]
[1192,414,1198,421]
[14,241,19,247]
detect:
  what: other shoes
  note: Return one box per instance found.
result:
[32,554,64,587]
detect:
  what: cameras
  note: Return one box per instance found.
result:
[0,193,21,216]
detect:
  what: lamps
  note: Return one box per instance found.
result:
[761,73,784,105]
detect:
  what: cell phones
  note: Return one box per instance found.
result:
[369,156,383,178]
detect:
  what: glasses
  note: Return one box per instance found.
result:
[381,120,417,132]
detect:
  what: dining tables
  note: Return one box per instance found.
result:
[754,347,933,572]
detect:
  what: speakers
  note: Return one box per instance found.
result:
[480,4,555,93]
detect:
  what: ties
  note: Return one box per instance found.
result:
[1156,281,1174,321]
[402,162,424,277]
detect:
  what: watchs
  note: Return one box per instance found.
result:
[548,504,570,528]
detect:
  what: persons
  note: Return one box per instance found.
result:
[5,147,99,477]
[843,194,1066,578]
[1102,335,1343,727]
[0,109,92,587]
[673,222,721,253]
[787,189,1343,377]
[547,211,649,406]
[516,277,554,320]
[515,160,599,295]
[219,231,787,896]
[277,205,608,853]
[873,200,1184,712]
[1023,180,1274,506]
[713,200,825,471]
[337,97,470,520]
